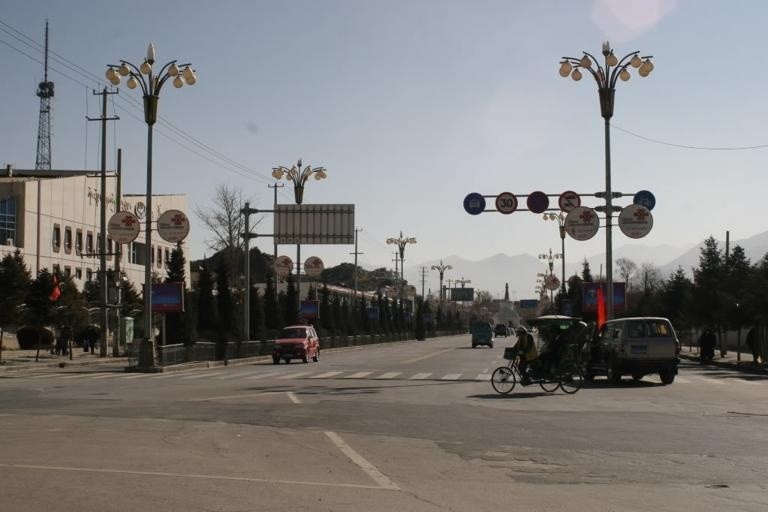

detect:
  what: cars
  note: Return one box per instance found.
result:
[469,320,536,348]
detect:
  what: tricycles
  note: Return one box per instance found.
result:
[491,343,584,396]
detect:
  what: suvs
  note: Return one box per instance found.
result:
[271,324,322,366]
[583,315,680,386]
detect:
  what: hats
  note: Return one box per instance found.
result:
[517,326,527,333]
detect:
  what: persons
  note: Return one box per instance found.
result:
[152,324,161,346]
[513,325,538,386]
[539,321,589,378]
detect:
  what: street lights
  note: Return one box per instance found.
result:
[557,39,655,319]
[431,259,453,332]
[541,208,570,316]
[455,276,472,310]
[538,247,564,316]
[271,156,329,326]
[105,41,198,374]
[534,269,550,316]
[385,230,418,341]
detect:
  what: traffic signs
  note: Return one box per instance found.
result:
[496,192,519,214]
